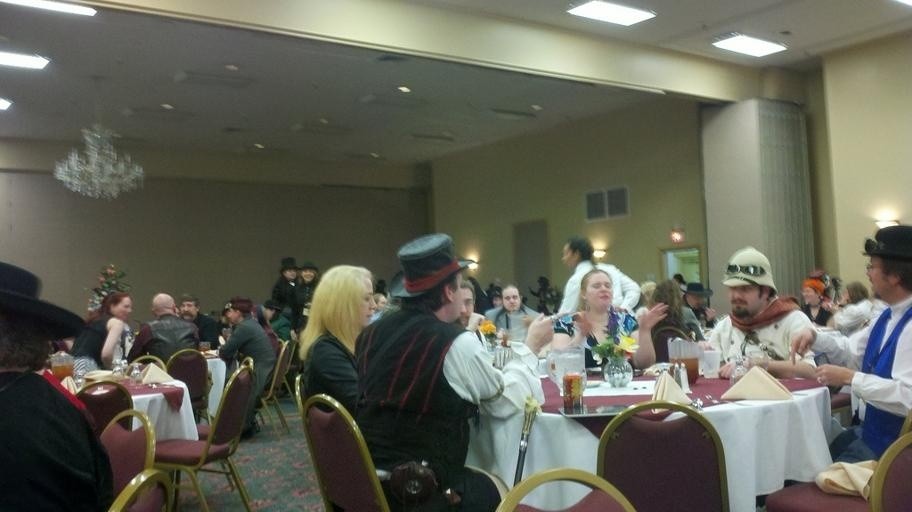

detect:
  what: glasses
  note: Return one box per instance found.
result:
[726,263,765,277]
[866,263,884,272]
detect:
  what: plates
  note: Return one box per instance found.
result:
[587,380,601,389]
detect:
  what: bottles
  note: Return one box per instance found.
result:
[730,348,747,387]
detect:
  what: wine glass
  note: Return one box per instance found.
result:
[111,356,144,388]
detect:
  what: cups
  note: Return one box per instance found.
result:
[200,341,209,353]
[51,351,74,382]
[703,350,720,379]
[749,351,769,373]
[668,338,699,385]
[545,345,586,391]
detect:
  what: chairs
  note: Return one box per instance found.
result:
[0,295,912,512]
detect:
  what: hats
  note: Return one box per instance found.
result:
[263,299,282,310]
[721,248,778,297]
[299,262,318,270]
[862,226,912,258]
[0,262,89,341]
[389,233,474,298]
[280,257,299,274]
[679,283,713,296]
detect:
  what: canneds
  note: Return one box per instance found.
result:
[562,373,582,410]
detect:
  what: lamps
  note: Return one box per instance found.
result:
[465,261,479,271]
[593,248,607,260]
[874,219,902,228]
[49,76,146,199]
